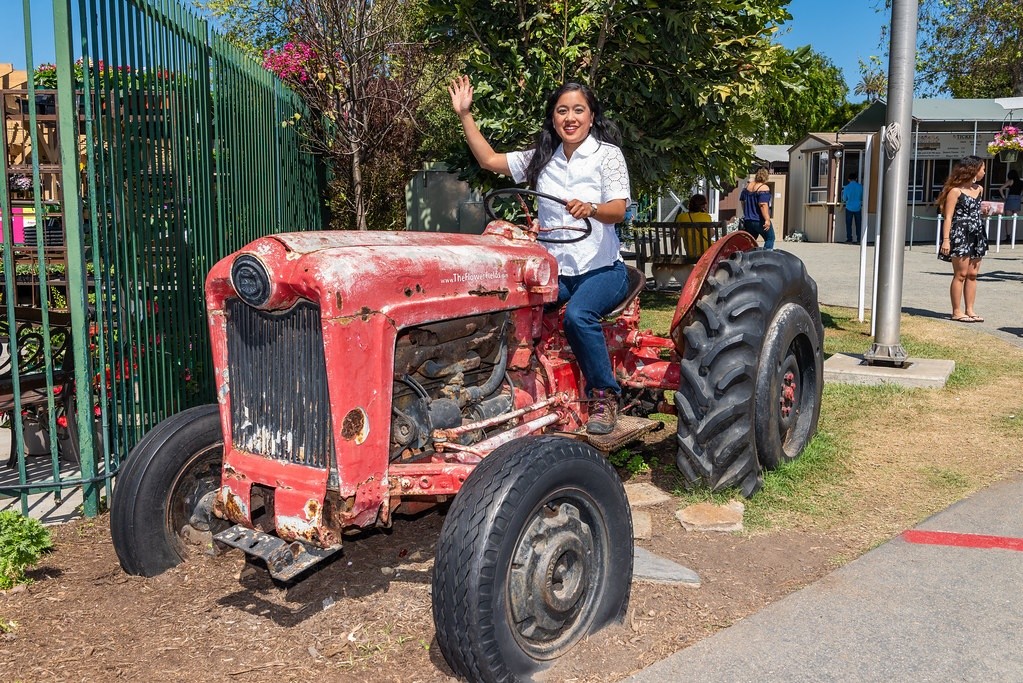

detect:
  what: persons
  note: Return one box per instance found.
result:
[739,167,776,248]
[935,156,989,322]
[448,75,631,434]
[673,194,715,258]
[843,172,863,245]
[623,210,634,234]
[999,169,1023,244]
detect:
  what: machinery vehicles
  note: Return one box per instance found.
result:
[111,186,825,683]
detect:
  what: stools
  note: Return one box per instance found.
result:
[606,265,645,320]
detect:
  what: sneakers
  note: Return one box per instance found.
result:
[586,387,618,434]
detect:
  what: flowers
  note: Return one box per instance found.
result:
[986,125,1023,156]
[33,55,182,93]
[0,303,166,429]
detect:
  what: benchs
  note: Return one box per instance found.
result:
[628,219,729,264]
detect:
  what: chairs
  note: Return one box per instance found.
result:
[0,305,90,471]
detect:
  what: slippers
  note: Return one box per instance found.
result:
[966,311,985,322]
[951,314,975,322]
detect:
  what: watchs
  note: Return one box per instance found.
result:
[588,201,597,217]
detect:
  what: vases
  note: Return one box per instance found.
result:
[20,417,57,453]
[60,417,108,464]
[1000,148,1019,162]
[17,93,176,114]
